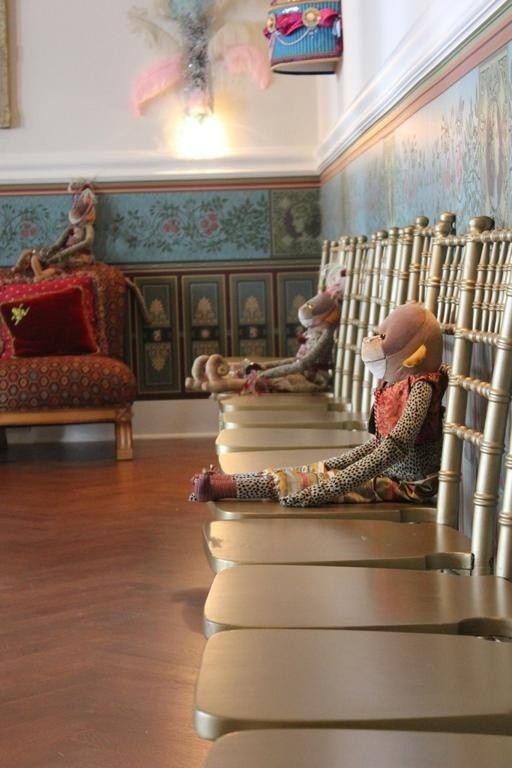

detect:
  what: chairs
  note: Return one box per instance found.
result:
[191,207,512,767]
[1,256,140,460]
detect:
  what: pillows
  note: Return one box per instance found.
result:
[0,270,111,362]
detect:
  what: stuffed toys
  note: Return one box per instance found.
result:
[190,299,449,509]
[184,279,345,395]
[14,189,97,287]
